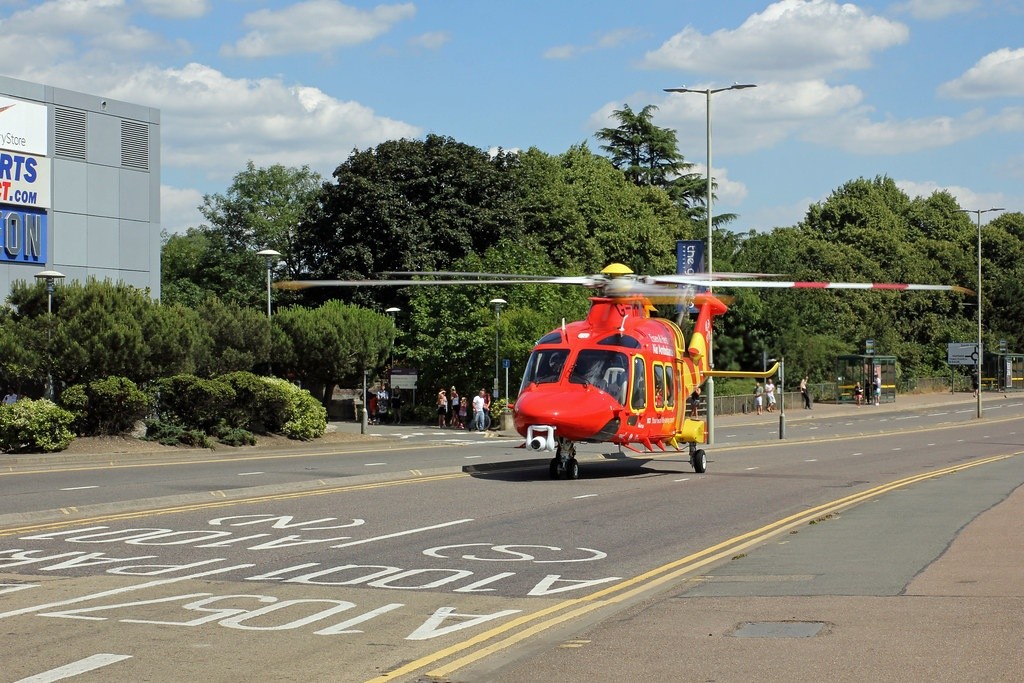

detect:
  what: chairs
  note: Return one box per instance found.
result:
[601,366,628,405]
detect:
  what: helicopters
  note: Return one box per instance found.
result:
[283,263,977,480]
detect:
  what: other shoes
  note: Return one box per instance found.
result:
[875,402,879,406]
[856,403,862,406]
[370,417,402,426]
[474,425,487,431]
[767,408,773,412]
[757,412,762,415]
[437,423,467,430]
[805,405,812,410]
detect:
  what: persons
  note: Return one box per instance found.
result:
[449,386,468,429]
[436,388,448,428]
[753,382,763,415]
[391,385,402,424]
[873,374,881,405]
[765,379,777,413]
[360,388,378,425]
[972,370,979,398]
[690,388,701,419]
[468,388,491,431]
[376,384,390,425]
[865,379,871,404]
[2,389,17,404]
[854,382,863,405]
[800,376,813,410]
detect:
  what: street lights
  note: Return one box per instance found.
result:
[663,84,759,446]
[489,298,508,428]
[34,271,65,399]
[386,307,401,369]
[255,249,282,376]
[951,209,1006,420]
